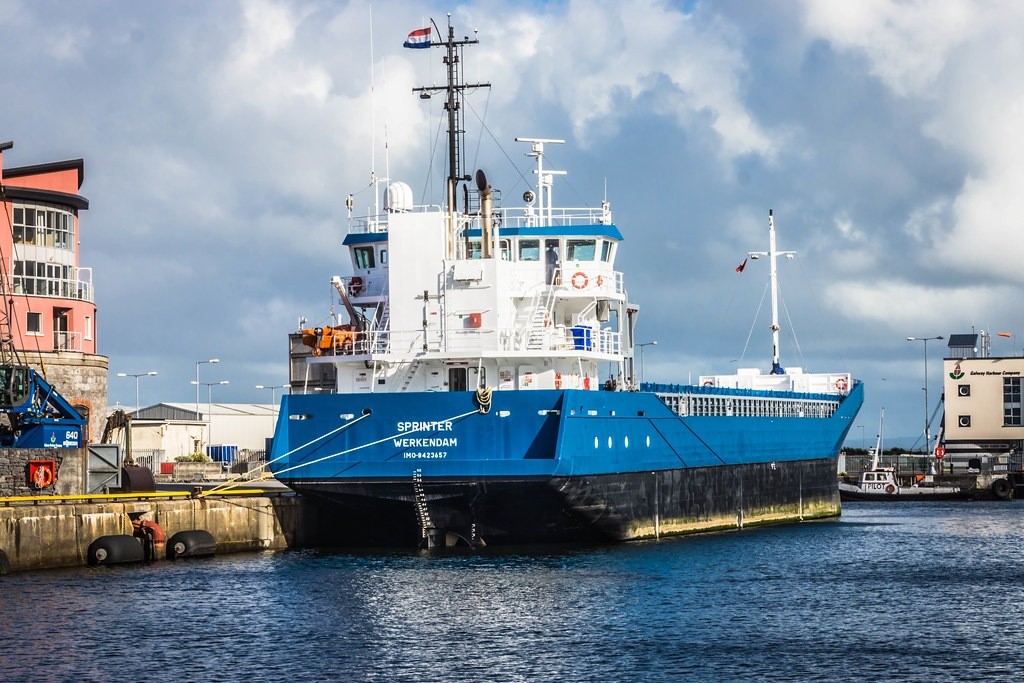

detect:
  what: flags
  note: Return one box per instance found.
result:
[736,258,748,274]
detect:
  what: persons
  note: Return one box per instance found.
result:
[221,461,229,473]
[844,473,851,485]
[546,244,558,285]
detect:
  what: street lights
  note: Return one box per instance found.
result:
[255,385,291,437]
[194,359,219,422]
[907,336,943,454]
[191,381,228,463]
[117,372,158,420]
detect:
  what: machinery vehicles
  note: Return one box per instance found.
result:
[0,137,86,449]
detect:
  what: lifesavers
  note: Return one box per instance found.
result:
[886,483,895,493]
[836,378,847,390]
[572,272,589,289]
[555,372,562,388]
[34,468,54,487]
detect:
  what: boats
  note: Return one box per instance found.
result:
[838,435,961,494]
[269,16,865,538]
